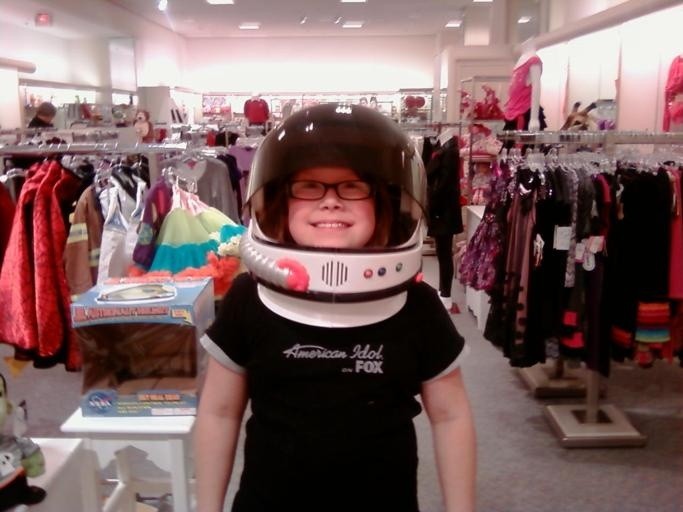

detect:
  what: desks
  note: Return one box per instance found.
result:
[0,408,199,511]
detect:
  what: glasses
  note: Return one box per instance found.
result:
[283,172,375,201]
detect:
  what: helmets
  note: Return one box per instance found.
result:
[238,101,428,327]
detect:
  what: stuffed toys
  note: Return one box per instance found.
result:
[132,110,153,139]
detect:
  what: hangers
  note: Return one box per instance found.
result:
[0,127,264,193]
[495,131,683,180]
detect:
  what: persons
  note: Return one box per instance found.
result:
[662,54,683,132]
[503,39,548,132]
[194,165,478,512]
[244,96,269,125]
[28,101,57,127]
[359,97,377,109]
[421,127,464,311]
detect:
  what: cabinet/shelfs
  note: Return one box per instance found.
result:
[465,205,491,333]
